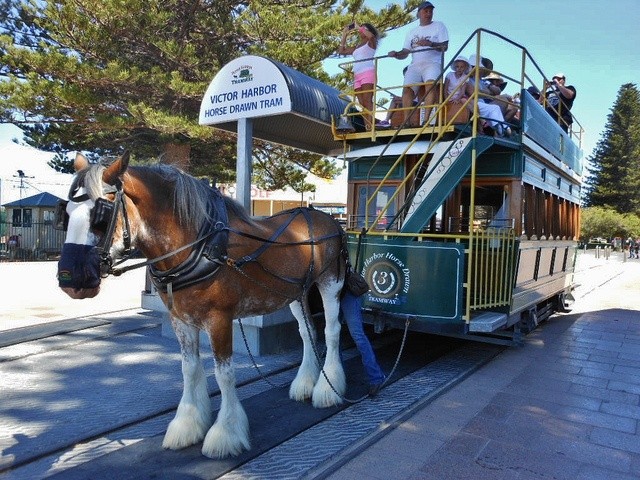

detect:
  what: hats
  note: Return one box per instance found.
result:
[552,73,566,79]
[495,79,507,91]
[418,2,435,12]
[451,56,472,73]
[469,55,493,76]
[482,71,502,80]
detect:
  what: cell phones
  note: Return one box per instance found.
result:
[348,23,355,32]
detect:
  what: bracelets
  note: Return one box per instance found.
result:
[340,44,346,46]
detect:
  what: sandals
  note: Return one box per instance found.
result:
[399,123,412,129]
[422,121,430,127]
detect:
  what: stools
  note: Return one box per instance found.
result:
[391,107,420,129]
[442,100,471,124]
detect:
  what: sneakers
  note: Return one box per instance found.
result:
[368,376,386,398]
[375,120,390,127]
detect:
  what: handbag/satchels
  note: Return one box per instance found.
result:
[347,271,369,297]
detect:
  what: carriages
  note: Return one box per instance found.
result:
[57,28,585,462]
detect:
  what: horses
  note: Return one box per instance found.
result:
[55,148,348,461]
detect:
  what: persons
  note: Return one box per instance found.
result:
[479,54,521,138]
[340,20,378,132]
[338,273,387,398]
[628,237,639,259]
[537,74,576,134]
[375,66,419,127]
[444,56,489,135]
[388,1,449,129]
